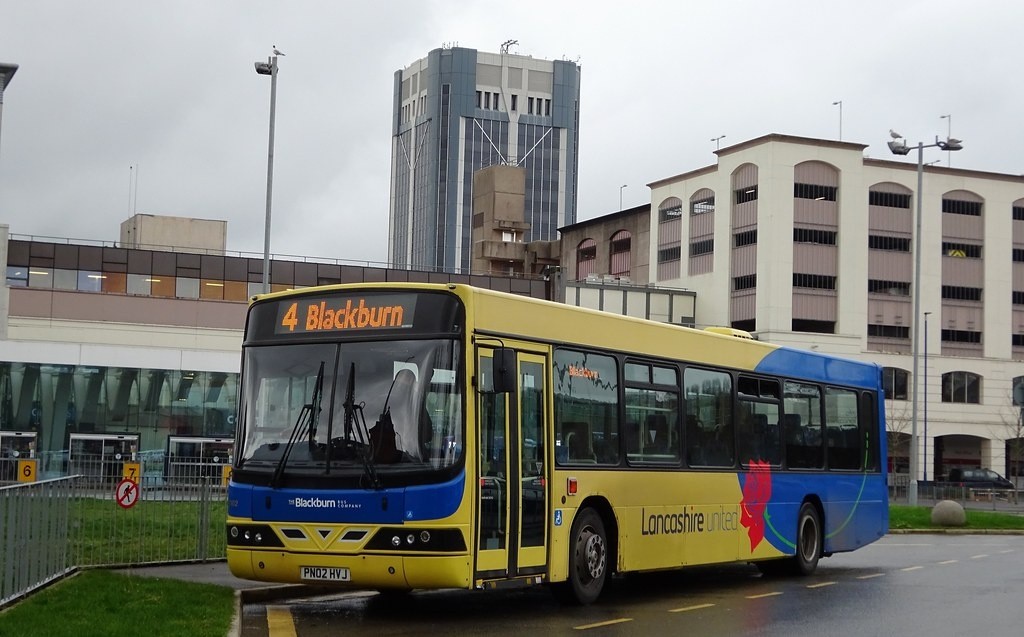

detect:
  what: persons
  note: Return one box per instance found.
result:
[374,369,433,462]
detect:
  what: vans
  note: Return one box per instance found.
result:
[948,467,1016,498]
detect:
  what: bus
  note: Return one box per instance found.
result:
[224,279,890,604]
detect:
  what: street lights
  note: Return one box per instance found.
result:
[940,115,951,167]
[832,101,843,141]
[618,183,627,212]
[923,312,933,487]
[709,135,727,150]
[887,137,963,506]
[254,45,285,294]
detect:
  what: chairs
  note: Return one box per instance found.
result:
[560,412,859,469]
